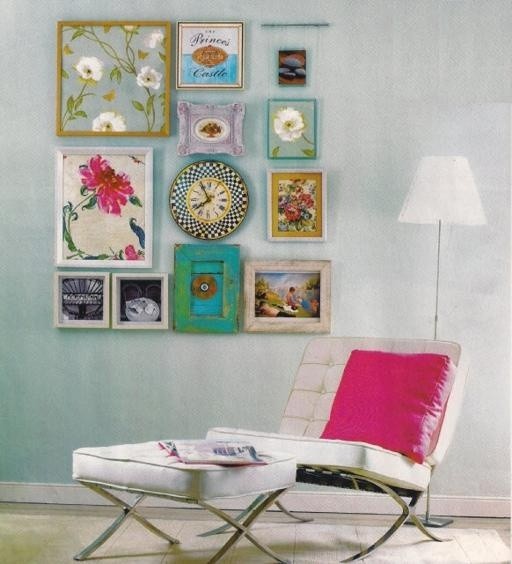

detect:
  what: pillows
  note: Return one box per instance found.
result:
[319,348,457,465]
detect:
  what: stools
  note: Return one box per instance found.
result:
[71,440,298,564]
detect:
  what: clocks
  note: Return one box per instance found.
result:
[169,160,248,241]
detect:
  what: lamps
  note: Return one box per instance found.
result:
[398,156,490,527]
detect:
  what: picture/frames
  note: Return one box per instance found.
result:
[265,98,317,161]
[176,19,243,91]
[111,272,169,330]
[53,145,154,269]
[172,243,240,334]
[56,18,173,139]
[266,169,327,243]
[53,271,111,329]
[177,100,246,157]
[261,22,319,98]
[243,257,333,333]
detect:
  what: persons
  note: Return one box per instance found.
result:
[287,287,303,311]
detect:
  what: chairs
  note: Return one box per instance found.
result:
[199,336,469,564]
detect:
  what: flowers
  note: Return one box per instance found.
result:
[269,101,314,157]
[63,24,165,132]
[277,178,316,232]
[64,154,146,261]
